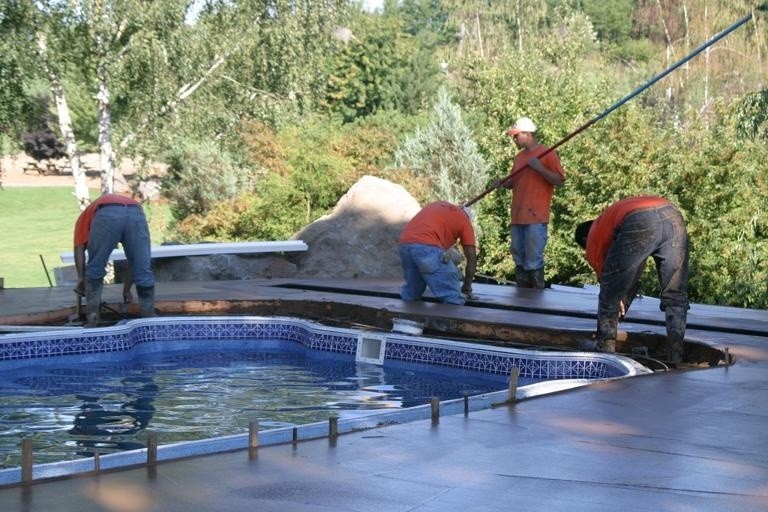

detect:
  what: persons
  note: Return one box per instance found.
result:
[73,193,159,326]
[486,116,565,289]
[574,193,689,365]
[397,199,477,307]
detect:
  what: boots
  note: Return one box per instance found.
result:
[514,266,543,289]
[84,278,102,327]
[580,299,618,353]
[657,306,686,362]
[135,286,159,317]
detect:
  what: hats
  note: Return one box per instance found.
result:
[505,117,535,135]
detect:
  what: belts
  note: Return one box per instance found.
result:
[94,203,141,212]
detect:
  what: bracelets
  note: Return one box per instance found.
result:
[460,286,472,293]
